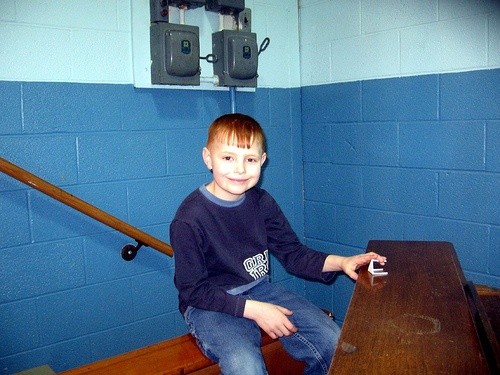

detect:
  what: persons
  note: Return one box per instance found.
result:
[169,113,387,375]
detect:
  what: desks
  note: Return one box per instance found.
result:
[327,239,489,375]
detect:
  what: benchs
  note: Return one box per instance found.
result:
[55,307,334,375]
[467,281,500,375]
